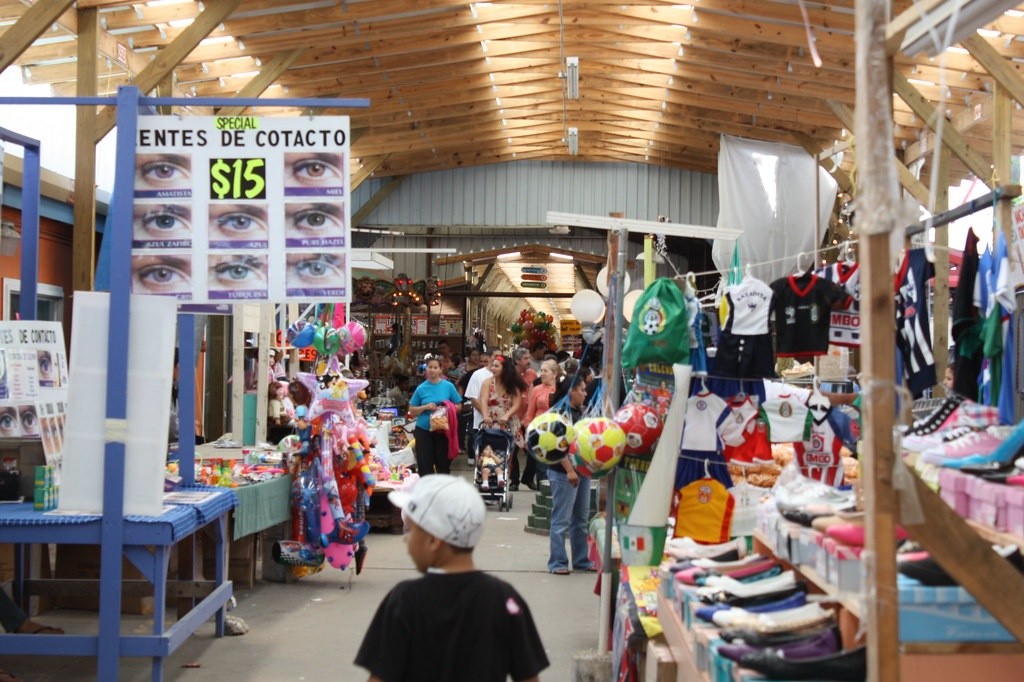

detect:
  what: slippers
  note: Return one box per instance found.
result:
[35,625,65,635]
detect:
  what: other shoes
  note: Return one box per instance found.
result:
[468,458,475,465]
[481,484,489,491]
[498,480,505,488]
[588,565,598,571]
[521,479,538,490]
[555,569,570,575]
[509,481,519,491]
[661,393,1022,682]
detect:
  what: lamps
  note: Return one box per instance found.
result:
[570,267,644,345]
[566,56,580,100]
[568,127,578,156]
[351,251,394,270]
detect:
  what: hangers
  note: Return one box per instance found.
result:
[837,240,856,266]
[685,271,698,298]
[734,379,747,404]
[777,375,791,396]
[806,376,831,412]
[792,251,808,280]
[701,458,713,480]
[697,372,713,395]
[744,262,756,282]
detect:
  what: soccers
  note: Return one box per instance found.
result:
[526,401,664,480]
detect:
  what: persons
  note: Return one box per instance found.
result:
[438,340,602,490]
[943,361,956,393]
[354,474,551,682]
[255,348,313,457]
[637,379,672,423]
[132,153,344,292]
[805,376,863,443]
[407,354,463,477]
[38,353,54,386]
[548,377,599,574]
[2,404,41,438]
[0,584,64,681]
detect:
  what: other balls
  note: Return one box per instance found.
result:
[287,319,367,356]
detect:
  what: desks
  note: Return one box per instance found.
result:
[0,483,241,682]
[29,473,293,621]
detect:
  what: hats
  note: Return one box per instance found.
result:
[387,474,486,548]
[424,353,434,360]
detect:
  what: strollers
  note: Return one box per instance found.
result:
[472,420,513,512]
